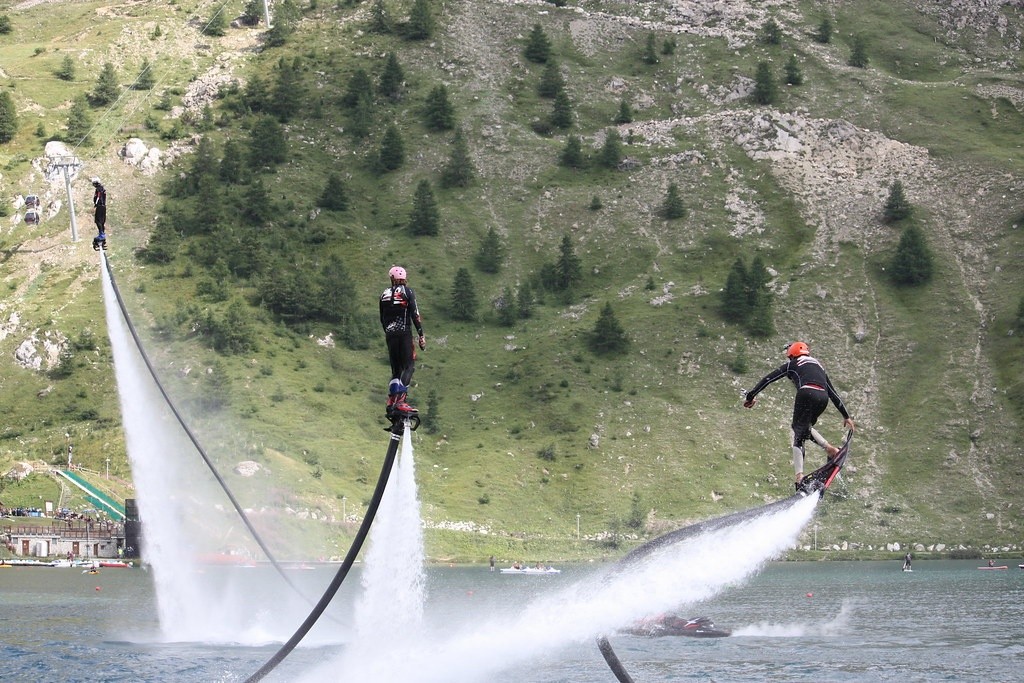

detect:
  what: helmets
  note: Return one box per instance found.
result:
[389,266,407,280]
[92,178,100,184]
[787,342,810,359]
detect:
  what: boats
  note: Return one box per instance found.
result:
[101,563,129,567]
[89,569,99,575]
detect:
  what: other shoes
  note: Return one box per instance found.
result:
[827,448,840,463]
[392,390,419,413]
[385,394,395,412]
[94,231,106,241]
[795,481,802,491]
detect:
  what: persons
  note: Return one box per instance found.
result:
[0,506,127,532]
[117,546,134,558]
[222,546,249,558]
[67,551,74,561]
[906,552,913,569]
[489,554,541,572]
[91,181,108,238]
[988,559,996,566]
[381,266,425,420]
[746,342,856,489]
